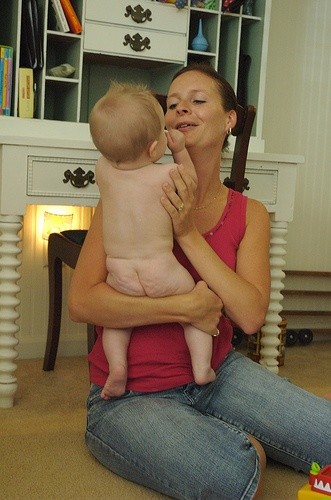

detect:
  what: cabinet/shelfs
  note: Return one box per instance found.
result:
[0,1,305,409]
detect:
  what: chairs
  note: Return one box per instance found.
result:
[41,95,257,386]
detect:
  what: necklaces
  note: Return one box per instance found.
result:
[192,181,223,210]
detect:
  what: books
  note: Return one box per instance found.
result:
[0,0,83,119]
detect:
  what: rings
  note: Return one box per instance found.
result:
[179,203,184,212]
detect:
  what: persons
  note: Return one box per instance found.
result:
[68,63,331,500]
[90,82,218,401]
